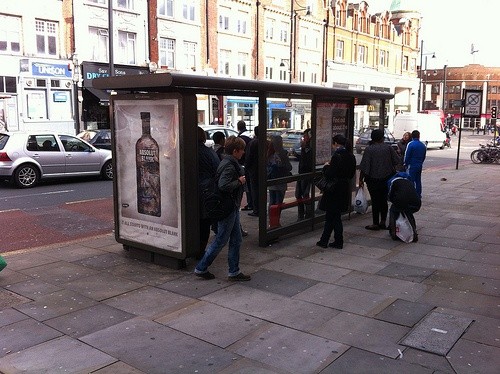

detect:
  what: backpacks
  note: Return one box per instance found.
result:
[404,188,421,212]
[198,162,242,221]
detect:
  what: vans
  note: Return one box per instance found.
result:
[417,109,446,133]
[392,111,446,150]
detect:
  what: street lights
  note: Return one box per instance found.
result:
[416,40,439,111]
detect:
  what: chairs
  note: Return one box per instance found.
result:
[43,140,52,150]
[29,139,40,150]
[61,140,67,148]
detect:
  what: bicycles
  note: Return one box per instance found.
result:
[470,136,500,165]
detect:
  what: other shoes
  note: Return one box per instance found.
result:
[241,205,252,210]
[380,223,387,229]
[317,241,328,248]
[329,242,343,249]
[193,270,215,278]
[242,230,248,236]
[228,273,251,281]
[248,210,258,216]
[211,225,219,234]
[365,224,380,231]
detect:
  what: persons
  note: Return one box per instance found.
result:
[265,136,293,228]
[315,135,356,250]
[445,121,494,148]
[193,121,427,282]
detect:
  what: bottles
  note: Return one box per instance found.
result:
[136,112,161,217]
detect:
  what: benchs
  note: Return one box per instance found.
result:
[269,193,323,229]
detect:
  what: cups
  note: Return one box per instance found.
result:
[290,148,295,156]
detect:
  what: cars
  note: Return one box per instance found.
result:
[355,127,395,154]
[72,129,112,150]
[0,131,112,189]
[266,127,305,158]
[198,124,239,150]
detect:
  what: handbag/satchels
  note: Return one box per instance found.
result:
[312,171,330,194]
[269,162,291,179]
[395,212,413,243]
[353,188,369,214]
